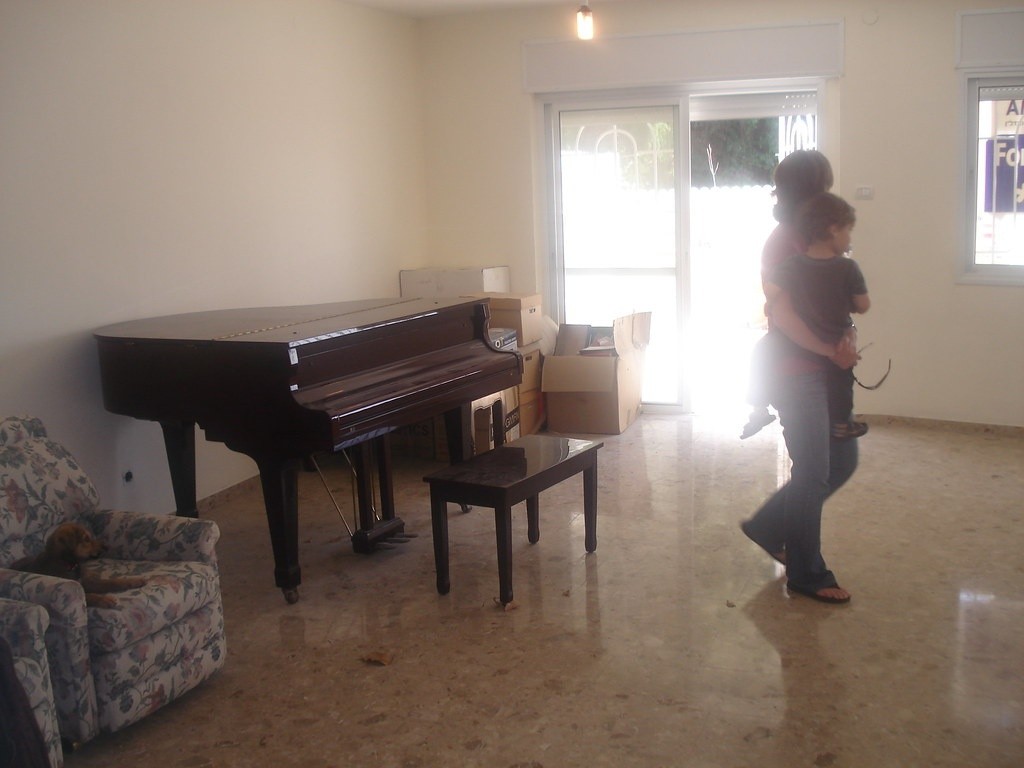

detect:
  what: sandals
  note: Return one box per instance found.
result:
[739,409,776,440]
[833,422,868,441]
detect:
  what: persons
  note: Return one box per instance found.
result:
[739,193,868,442]
[741,149,859,605]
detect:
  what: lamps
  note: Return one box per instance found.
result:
[576,0,594,40]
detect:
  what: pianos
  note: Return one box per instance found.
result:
[91,294,526,605]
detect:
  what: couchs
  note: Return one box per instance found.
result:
[0,413,228,768]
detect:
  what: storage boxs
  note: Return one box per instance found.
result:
[400,267,653,461]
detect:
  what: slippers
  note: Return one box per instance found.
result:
[787,570,850,603]
[741,521,786,564]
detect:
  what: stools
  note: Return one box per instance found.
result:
[422,434,605,606]
